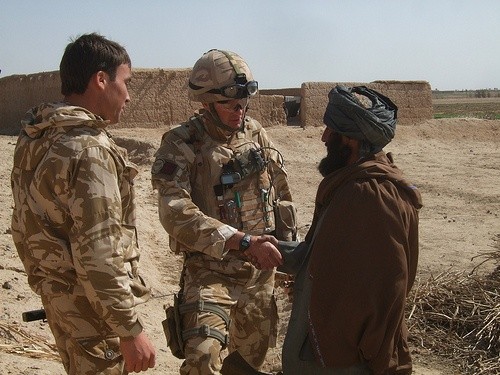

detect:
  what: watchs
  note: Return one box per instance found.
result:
[239,233,251,253]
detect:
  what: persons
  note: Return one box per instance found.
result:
[11,32,156,375]
[244,86,423,375]
[151,49,297,375]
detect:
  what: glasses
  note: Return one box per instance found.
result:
[220,81,258,98]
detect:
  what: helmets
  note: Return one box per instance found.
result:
[188,49,253,102]
